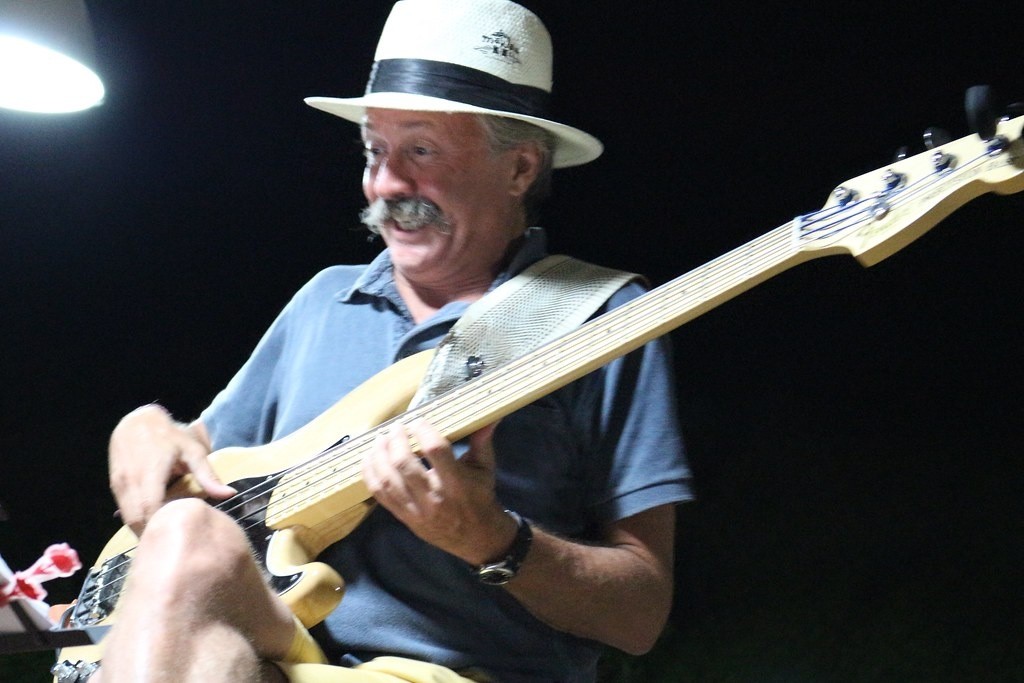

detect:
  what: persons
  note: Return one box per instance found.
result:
[86,0,695,683]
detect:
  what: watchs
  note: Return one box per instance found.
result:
[468,510,533,586]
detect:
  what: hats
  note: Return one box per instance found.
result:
[303,0,604,169]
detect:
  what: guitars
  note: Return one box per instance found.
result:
[47,86,1024,683]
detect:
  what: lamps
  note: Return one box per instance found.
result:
[0,0,107,116]
